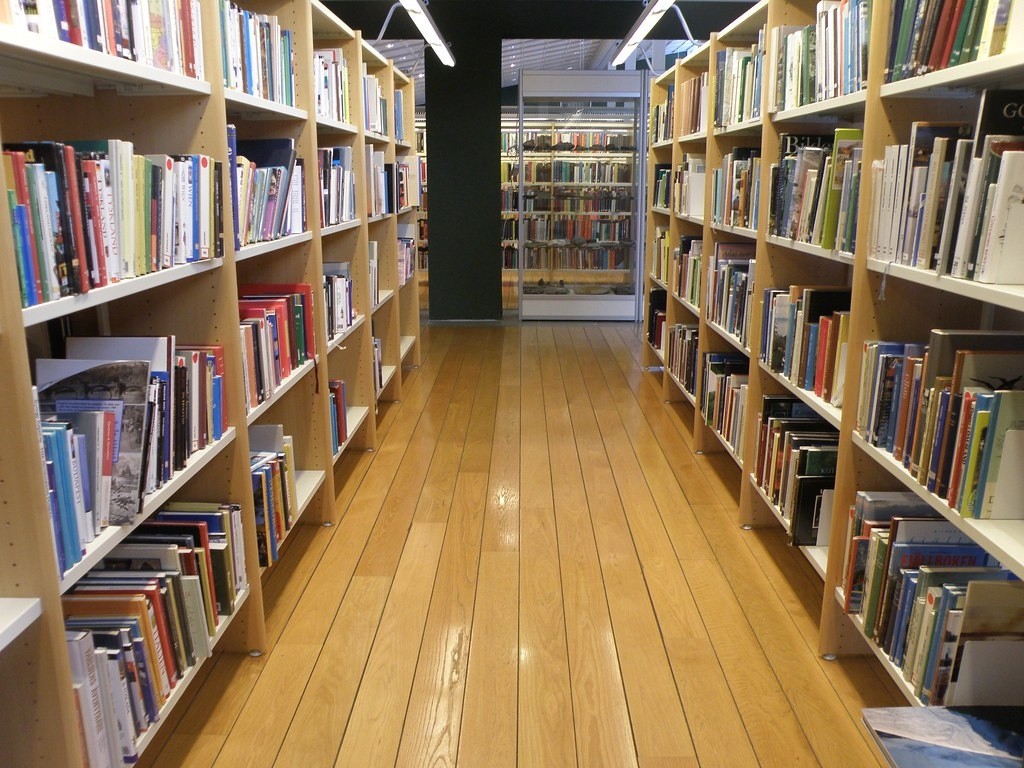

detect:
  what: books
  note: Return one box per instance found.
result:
[219,0,295,107]
[238,284,316,415]
[317,146,355,228]
[368,241,380,309]
[66,501,248,768]
[329,379,347,457]
[32,334,228,580]
[361,63,639,286]
[228,124,309,250]
[372,337,384,397]
[647,0,1024,709]
[0,1,205,83]
[250,424,298,568]
[323,262,353,348]
[314,48,349,124]
[3,136,223,308]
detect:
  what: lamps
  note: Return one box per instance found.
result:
[371,0,457,77]
[611,0,703,77]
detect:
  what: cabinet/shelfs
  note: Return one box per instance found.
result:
[642,1,1024,767]
[0,0,422,768]
[414,70,650,320]
